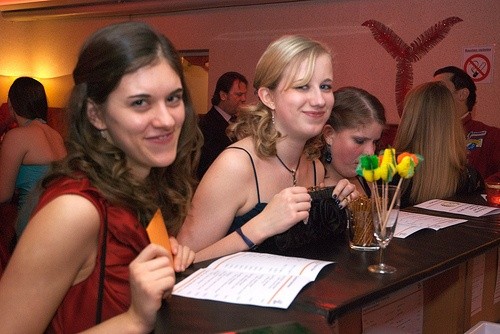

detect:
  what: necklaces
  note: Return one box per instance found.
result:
[272,143,302,189]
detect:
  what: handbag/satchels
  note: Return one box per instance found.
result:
[272,185,352,256]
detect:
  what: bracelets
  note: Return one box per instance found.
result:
[234,226,259,252]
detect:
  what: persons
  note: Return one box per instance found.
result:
[0,20,500,334]
[0,20,205,334]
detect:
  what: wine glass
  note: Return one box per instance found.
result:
[367,185,401,274]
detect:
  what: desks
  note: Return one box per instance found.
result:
[154,192,500,334]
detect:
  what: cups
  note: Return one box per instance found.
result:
[347,203,381,252]
